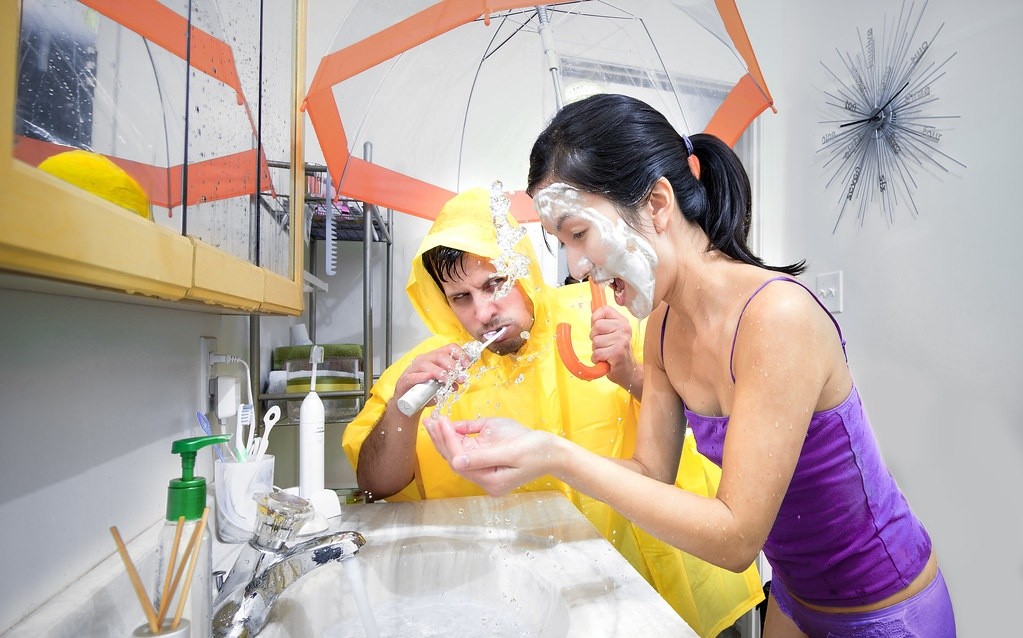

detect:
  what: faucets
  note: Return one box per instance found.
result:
[207,490,365,638]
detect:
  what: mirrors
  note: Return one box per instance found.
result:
[12,1,304,281]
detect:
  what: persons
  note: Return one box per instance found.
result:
[339,190,766,638]
[415,91,958,638]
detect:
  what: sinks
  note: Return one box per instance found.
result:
[226,527,623,638]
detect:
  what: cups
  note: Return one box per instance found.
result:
[216,455,275,542]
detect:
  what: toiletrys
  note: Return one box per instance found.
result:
[141,434,236,638]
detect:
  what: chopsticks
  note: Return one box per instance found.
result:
[101,506,211,630]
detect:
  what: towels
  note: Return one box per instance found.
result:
[269,340,366,362]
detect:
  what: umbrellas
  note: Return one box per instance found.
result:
[303,3,782,386]
[11,2,279,222]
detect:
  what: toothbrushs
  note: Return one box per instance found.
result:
[297,342,327,498]
[395,324,507,418]
[194,411,230,462]
[232,404,252,464]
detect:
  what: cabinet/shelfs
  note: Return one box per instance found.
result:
[0,0,311,324]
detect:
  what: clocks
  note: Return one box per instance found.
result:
[818,3,965,235]
